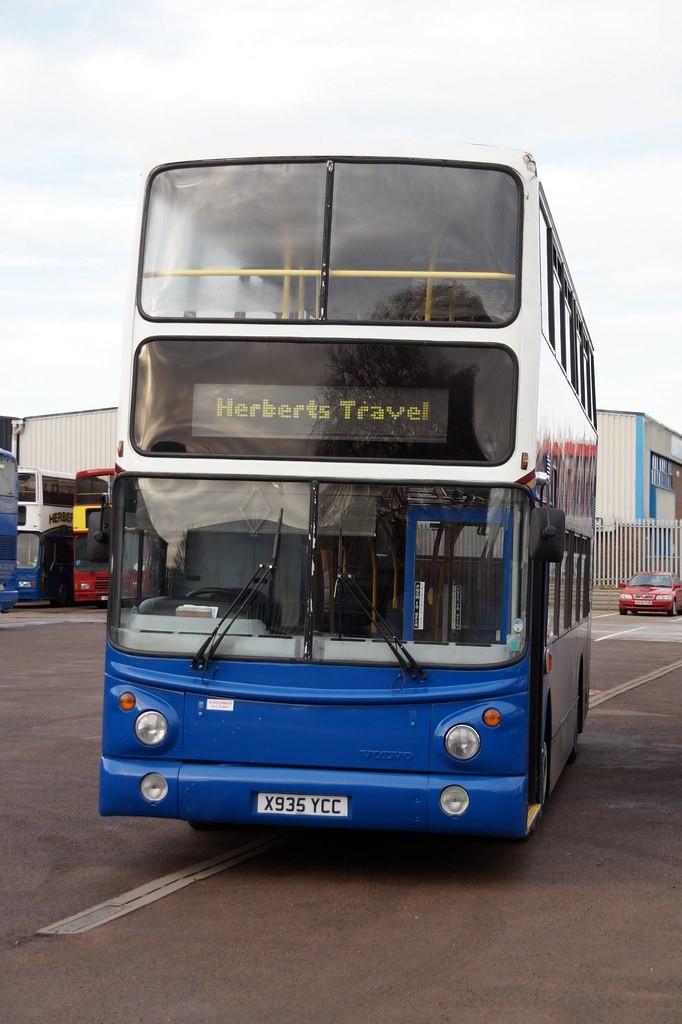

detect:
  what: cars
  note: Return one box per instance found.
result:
[618,570,682,616]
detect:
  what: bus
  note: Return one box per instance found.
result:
[0,448,20,613]
[75,467,167,610]
[13,465,108,607]
[87,149,596,844]
